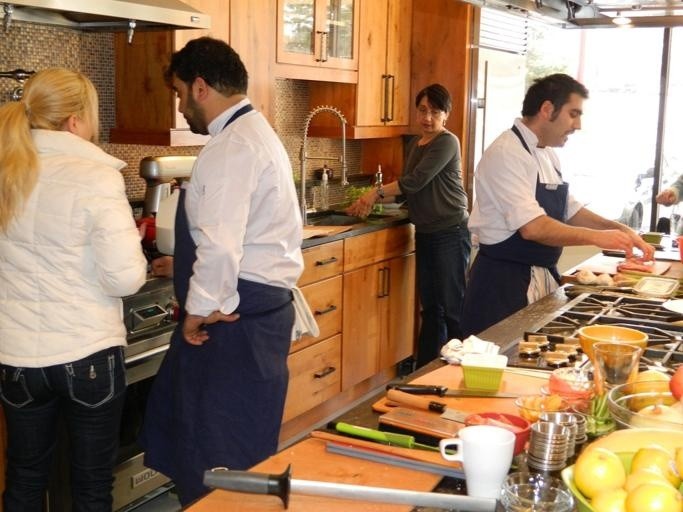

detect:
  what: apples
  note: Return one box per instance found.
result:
[573,447,683,512]
[629,370,677,410]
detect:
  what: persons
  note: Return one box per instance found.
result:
[0,69,148,510]
[141,37,305,511]
[346,82,471,368]
[460,73,656,344]
[657,174,683,206]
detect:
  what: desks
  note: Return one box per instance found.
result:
[563,229,682,301]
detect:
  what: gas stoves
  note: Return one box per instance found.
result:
[500,290,682,379]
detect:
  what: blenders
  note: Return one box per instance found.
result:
[140,157,197,243]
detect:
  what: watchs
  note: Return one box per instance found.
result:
[377,186,385,199]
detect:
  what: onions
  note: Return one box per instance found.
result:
[630,405,683,430]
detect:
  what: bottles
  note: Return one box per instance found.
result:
[373,165,383,211]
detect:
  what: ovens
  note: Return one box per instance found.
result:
[108,284,201,510]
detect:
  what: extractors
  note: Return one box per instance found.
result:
[0,0,212,30]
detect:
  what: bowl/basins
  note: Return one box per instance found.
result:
[577,325,648,365]
[465,412,530,453]
[606,381,682,432]
[458,356,506,393]
[546,369,595,399]
[574,401,614,439]
[514,394,569,422]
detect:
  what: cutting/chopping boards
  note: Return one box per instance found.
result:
[372,358,581,434]
[186,434,444,510]
[573,256,683,280]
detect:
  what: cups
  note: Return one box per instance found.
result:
[440,425,516,499]
[591,343,642,389]
[502,473,575,510]
[527,411,590,472]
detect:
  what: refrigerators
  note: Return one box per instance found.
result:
[467,47,533,209]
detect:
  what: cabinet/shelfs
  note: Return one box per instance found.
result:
[342,222,417,389]
[306,1,416,142]
[107,1,275,147]
[276,239,345,424]
[276,2,359,85]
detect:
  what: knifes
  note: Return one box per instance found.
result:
[325,420,463,458]
[387,389,524,434]
[384,382,520,400]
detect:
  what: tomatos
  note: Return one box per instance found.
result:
[669,366,683,401]
